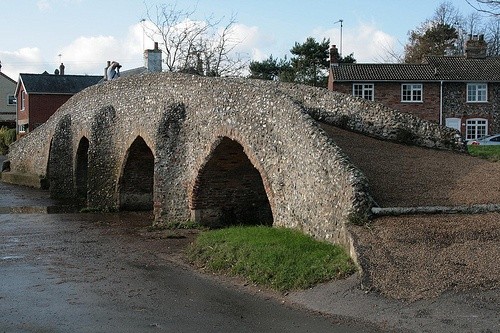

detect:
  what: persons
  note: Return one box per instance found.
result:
[107,61,122,81]
[104,61,111,79]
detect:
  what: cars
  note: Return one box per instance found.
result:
[468,135,500,145]
